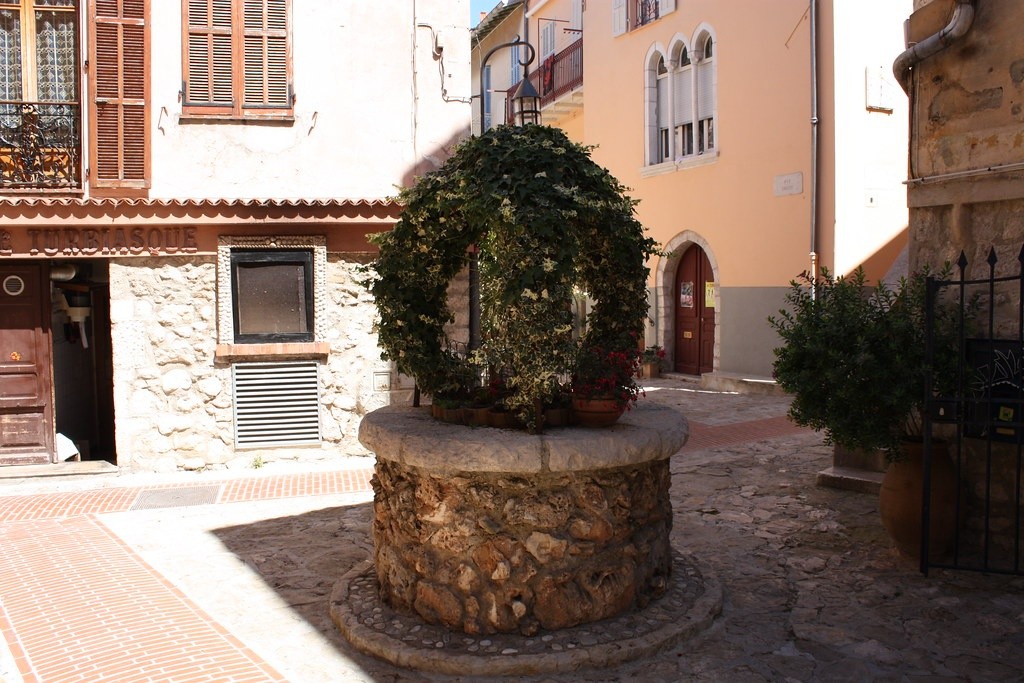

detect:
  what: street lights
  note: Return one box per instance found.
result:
[467,38,542,386]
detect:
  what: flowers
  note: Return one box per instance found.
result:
[559,330,667,411]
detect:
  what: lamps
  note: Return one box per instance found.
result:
[511,62,542,127]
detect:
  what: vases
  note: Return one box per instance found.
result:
[570,396,627,428]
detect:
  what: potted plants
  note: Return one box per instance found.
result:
[432,387,513,428]
[767,266,1024,571]
